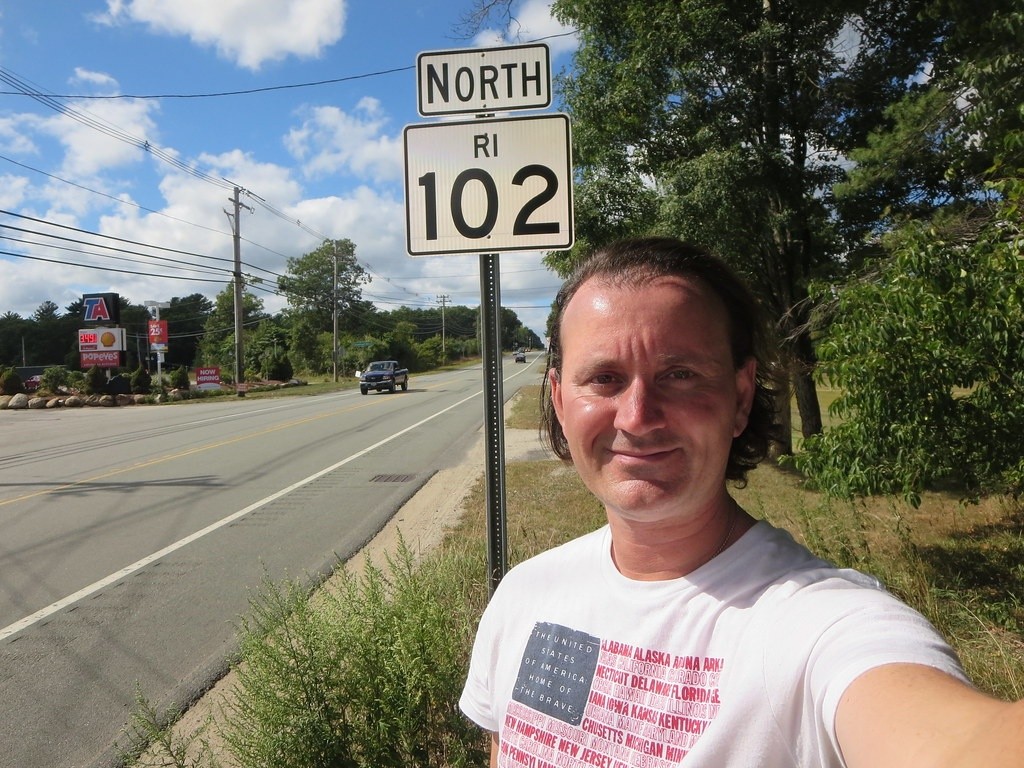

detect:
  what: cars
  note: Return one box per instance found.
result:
[25,376,40,389]
[359,361,409,395]
[515,353,526,363]
[513,347,531,356]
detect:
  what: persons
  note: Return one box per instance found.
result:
[459,236,1024,768]
